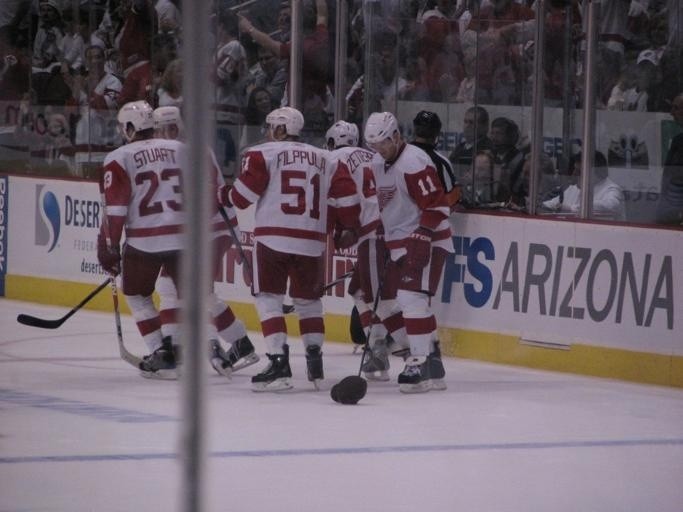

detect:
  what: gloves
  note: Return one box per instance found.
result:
[216,185,233,208]
[334,228,359,249]
[403,227,431,265]
[98,233,122,275]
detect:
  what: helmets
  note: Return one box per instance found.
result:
[265,107,441,146]
[116,100,183,141]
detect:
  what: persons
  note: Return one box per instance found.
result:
[403,110,459,364]
[153,105,259,371]
[96,100,225,378]
[363,112,453,387]
[1,1,683,222]
[211,108,361,385]
[324,120,411,364]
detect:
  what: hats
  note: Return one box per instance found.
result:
[637,49,658,67]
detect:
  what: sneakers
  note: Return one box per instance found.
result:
[362,338,444,384]
[140,335,323,382]
